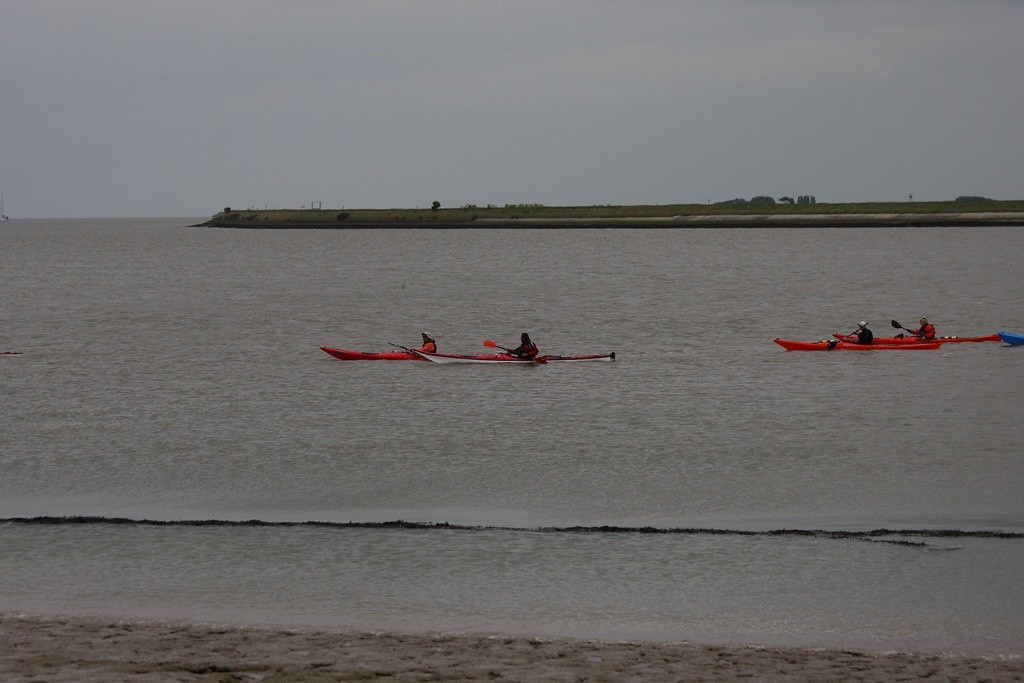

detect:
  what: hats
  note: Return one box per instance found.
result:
[421,331,434,341]
[858,321,866,328]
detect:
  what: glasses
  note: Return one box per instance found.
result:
[422,336,427,338]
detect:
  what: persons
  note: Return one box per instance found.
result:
[507,333,538,358]
[853,321,873,345]
[417,331,437,353]
[912,316,936,339]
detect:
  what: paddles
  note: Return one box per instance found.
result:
[386,341,414,352]
[483,339,548,365]
[826,322,870,352]
[890,319,937,339]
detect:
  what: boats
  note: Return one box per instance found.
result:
[773,337,942,351]
[833,333,1002,343]
[320,345,428,361]
[998,331,1024,345]
[411,348,616,365]
